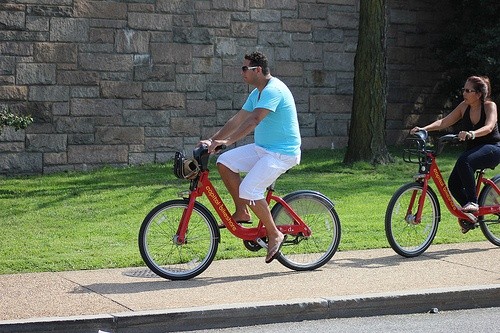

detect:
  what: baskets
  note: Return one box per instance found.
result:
[173,150,200,180]
[403,130,441,165]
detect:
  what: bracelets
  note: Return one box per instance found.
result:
[468,130,475,140]
[208,138,213,143]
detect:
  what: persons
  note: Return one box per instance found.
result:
[196,51,302,265]
[407,74,500,235]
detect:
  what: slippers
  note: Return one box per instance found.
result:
[265,235,288,263]
[219,212,253,228]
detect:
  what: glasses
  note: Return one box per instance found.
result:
[462,88,477,93]
[242,66,262,72]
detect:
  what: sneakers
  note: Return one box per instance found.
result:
[461,201,480,213]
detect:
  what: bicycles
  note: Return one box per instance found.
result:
[138,144,341,281]
[385,130,500,258]
